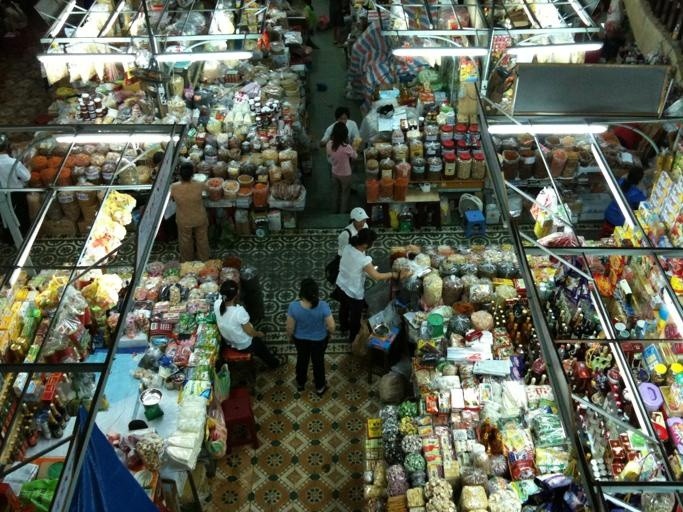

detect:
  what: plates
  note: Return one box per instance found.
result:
[140,387,163,407]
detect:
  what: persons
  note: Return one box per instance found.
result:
[321,107,360,196]
[214,279,287,369]
[604,166,647,226]
[650,97,683,157]
[0,134,31,244]
[338,207,370,258]
[288,277,335,394]
[171,163,211,262]
[336,228,399,341]
[325,123,358,214]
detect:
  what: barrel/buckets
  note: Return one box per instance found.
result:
[427,313,444,337]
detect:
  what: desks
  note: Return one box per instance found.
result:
[343,2,633,234]
[20,3,312,234]
[362,244,590,510]
[3,259,230,511]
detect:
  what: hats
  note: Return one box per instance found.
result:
[349,207,370,222]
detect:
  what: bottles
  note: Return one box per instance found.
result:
[487,302,630,451]
[0,347,85,464]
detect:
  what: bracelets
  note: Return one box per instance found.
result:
[392,271,395,279]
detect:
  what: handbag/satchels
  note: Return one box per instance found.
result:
[324,229,352,285]
